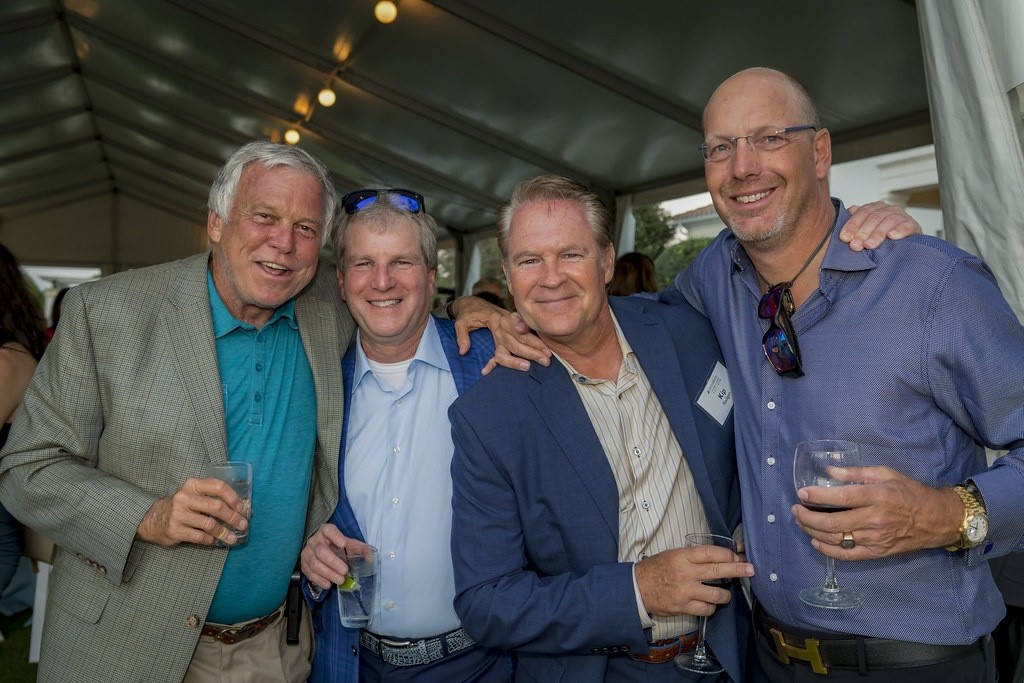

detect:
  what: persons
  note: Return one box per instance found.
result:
[494,66,1024,683]
[0,142,510,683]
[607,252,659,295]
[301,192,921,683]
[0,244,70,683]
[472,278,507,308]
[449,175,745,683]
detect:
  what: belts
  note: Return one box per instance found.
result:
[201,611,282,645]
[751,595,991,675]
[359,625,476,667]
[626,631,701,665]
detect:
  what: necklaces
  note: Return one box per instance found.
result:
[756,205,839,318]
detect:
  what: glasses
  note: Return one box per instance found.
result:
[340,188,426,216]
[699,123,818,162]
[758,280,805,380]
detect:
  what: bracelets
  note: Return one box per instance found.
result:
[447,300,457,320]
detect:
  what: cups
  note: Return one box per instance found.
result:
[209,461,252,547]
[336,547,378,629]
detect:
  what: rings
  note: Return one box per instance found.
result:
[841,532,856,549]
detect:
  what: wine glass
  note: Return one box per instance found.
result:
[674,534,737,673]
[793,441,866,610]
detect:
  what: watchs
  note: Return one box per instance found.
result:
[950,485,989,550]
